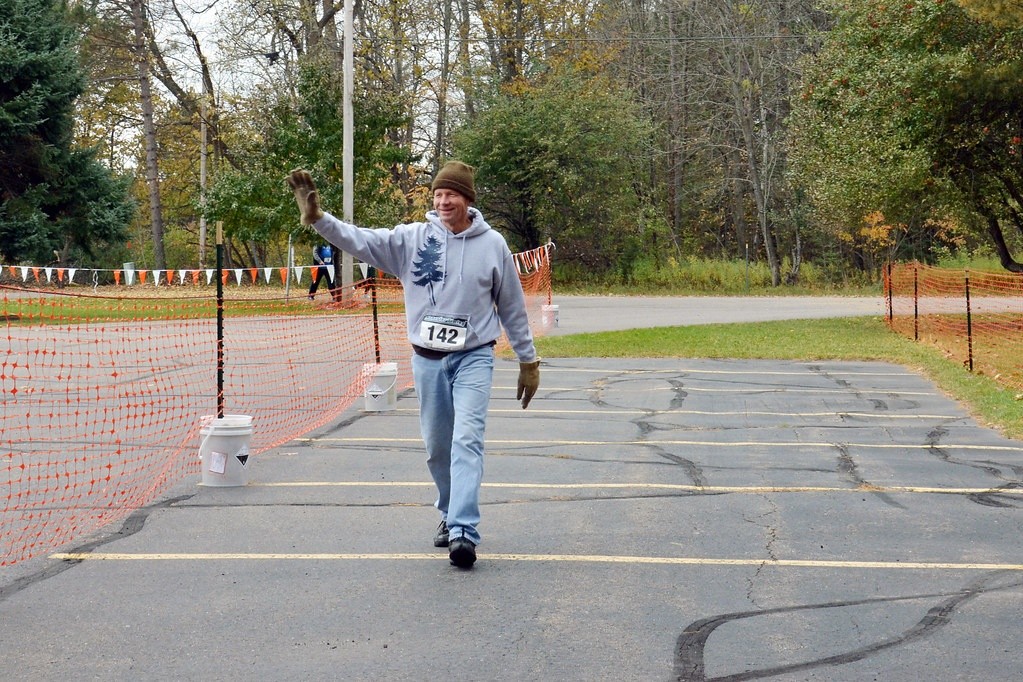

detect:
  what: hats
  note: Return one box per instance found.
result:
[434,161,477,202]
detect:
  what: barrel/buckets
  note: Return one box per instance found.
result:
[198,414,252,486]
[363,362,398,411]
[541,305,559,328]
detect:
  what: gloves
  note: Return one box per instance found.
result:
[517,356,542,409]
[286,168,324,226]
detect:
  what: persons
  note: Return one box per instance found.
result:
[357,260,375,295]
[289,161,540,568]
[308,239,342,303]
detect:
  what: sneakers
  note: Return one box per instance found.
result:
[433,521,450,547]
[448,537,476,565]
